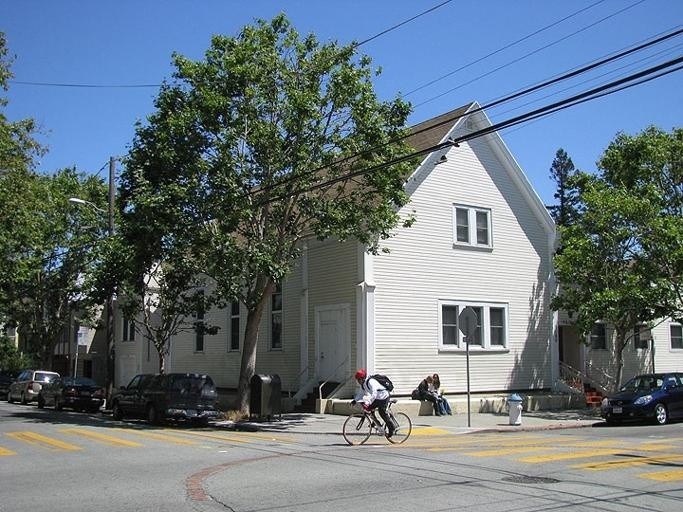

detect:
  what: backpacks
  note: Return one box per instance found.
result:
[361,374,393,392]
[412,389,423,400]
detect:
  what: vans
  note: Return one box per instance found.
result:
[7,370,61,405]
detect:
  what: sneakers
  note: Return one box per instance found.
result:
[435,410,452,416]
[368,420,398,438]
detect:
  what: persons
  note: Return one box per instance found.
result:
[347,370,397,440]
[428,374,452,416]
[418,375,444,416]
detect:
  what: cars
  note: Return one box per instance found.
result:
[599,371,683,426]
[36,375,105,413]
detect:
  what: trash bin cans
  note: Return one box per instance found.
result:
[249,373,281,423]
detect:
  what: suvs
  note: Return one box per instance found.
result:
[110,372,220,428]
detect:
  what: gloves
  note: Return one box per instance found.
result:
[350,400,356,406]
[361,403,369,409]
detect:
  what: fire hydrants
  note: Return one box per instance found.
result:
[508,393,523,426]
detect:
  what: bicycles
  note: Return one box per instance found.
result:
[342,398,412,447]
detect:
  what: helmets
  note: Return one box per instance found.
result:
[355,369,366,379]
